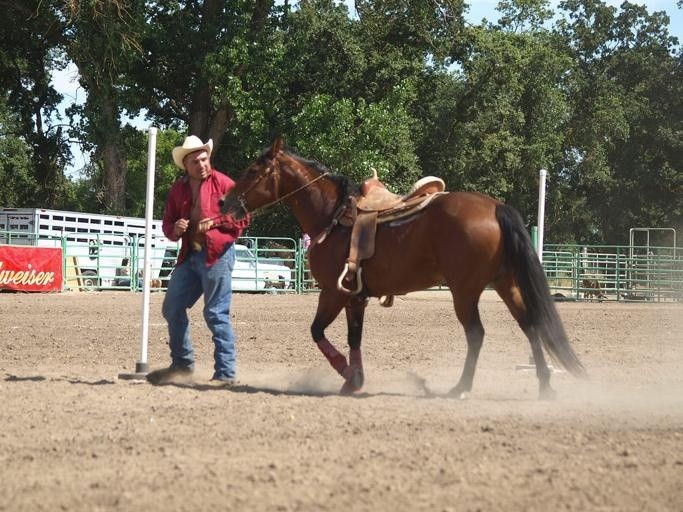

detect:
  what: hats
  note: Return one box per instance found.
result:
[171,135,214,170]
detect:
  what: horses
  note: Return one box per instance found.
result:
[264,241,316,289]
[216,130,587,402]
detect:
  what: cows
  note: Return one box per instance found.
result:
[583,279,607,303]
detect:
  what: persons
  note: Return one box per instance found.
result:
[151,134,251,384]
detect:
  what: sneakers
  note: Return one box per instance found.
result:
[147,367,196,385]
[194,379,240,388]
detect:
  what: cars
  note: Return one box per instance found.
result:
[160,244,291,294]
